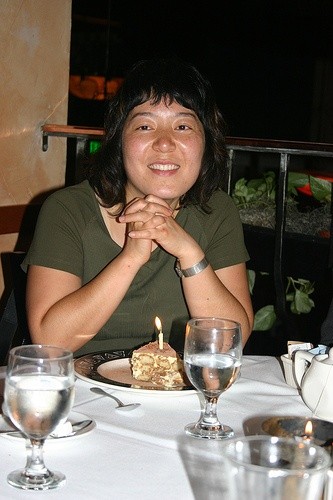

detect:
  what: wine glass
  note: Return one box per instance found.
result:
[5,346,75,490]
[184,317,243,440]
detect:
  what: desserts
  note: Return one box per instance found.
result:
[131,340,191,387]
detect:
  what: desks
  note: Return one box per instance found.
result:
[0,351,333,500]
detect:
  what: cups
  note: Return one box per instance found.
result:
[280,354,297,390]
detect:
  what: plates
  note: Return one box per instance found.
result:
[244,416,333,452]
[74,350,196,392]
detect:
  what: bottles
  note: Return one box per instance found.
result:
[180,435,331,500]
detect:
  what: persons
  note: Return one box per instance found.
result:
[19,66,254,359]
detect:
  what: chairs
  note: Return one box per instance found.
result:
[0,249,264,366]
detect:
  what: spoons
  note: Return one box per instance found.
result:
[92,387,140,413]
[0,420,96,438]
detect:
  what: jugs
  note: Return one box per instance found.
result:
[294,347,333,416]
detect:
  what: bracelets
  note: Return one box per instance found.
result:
[175,255,211,279]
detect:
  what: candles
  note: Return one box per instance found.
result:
[159,327,163,349]
